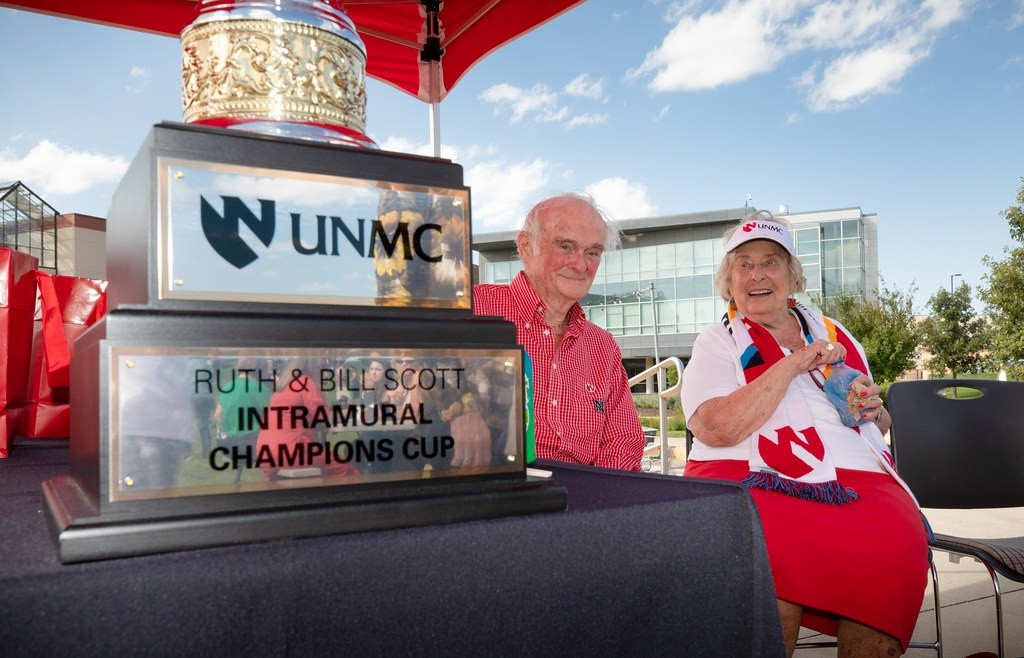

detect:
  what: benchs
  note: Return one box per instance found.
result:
[220,454,322,486]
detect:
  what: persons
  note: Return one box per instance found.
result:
[473,188,646,472]
[254,356,360,477]
[679,211,936,658]
[213,357,273,466]
[331,354,491,467]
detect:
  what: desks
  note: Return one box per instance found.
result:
[0,445,787,658]
[325,416,419,475]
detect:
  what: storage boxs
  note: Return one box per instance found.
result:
[41,119,570,558]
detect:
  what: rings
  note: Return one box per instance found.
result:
[826,343,835,352]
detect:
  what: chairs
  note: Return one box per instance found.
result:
[687,378,1024,658]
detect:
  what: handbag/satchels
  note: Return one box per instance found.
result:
[22,270,109,441]
[0,246,38,460]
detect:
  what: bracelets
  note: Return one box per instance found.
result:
[441,390,481,421]
[875,405,882,421]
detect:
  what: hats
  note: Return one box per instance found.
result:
[724,220,797,260]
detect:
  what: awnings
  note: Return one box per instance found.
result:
[0,0,590,194]
